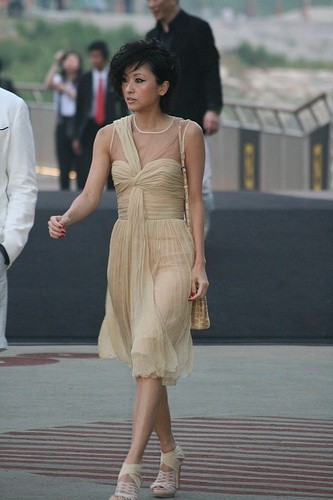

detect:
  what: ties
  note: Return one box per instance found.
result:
[96,77,105,125]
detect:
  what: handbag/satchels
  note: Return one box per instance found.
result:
[190,285,211,330]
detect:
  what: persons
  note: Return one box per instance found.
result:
[0,60,16,95]
[47,38,209,500]
[70,41,128,196]
[140,0,222,134]
[43,49,84,193]
[0,87,38,349]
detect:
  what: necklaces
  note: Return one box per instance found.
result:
[133,114,174,134]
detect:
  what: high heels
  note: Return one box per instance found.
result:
[150,445,184,497]
[109,463,143,500]
